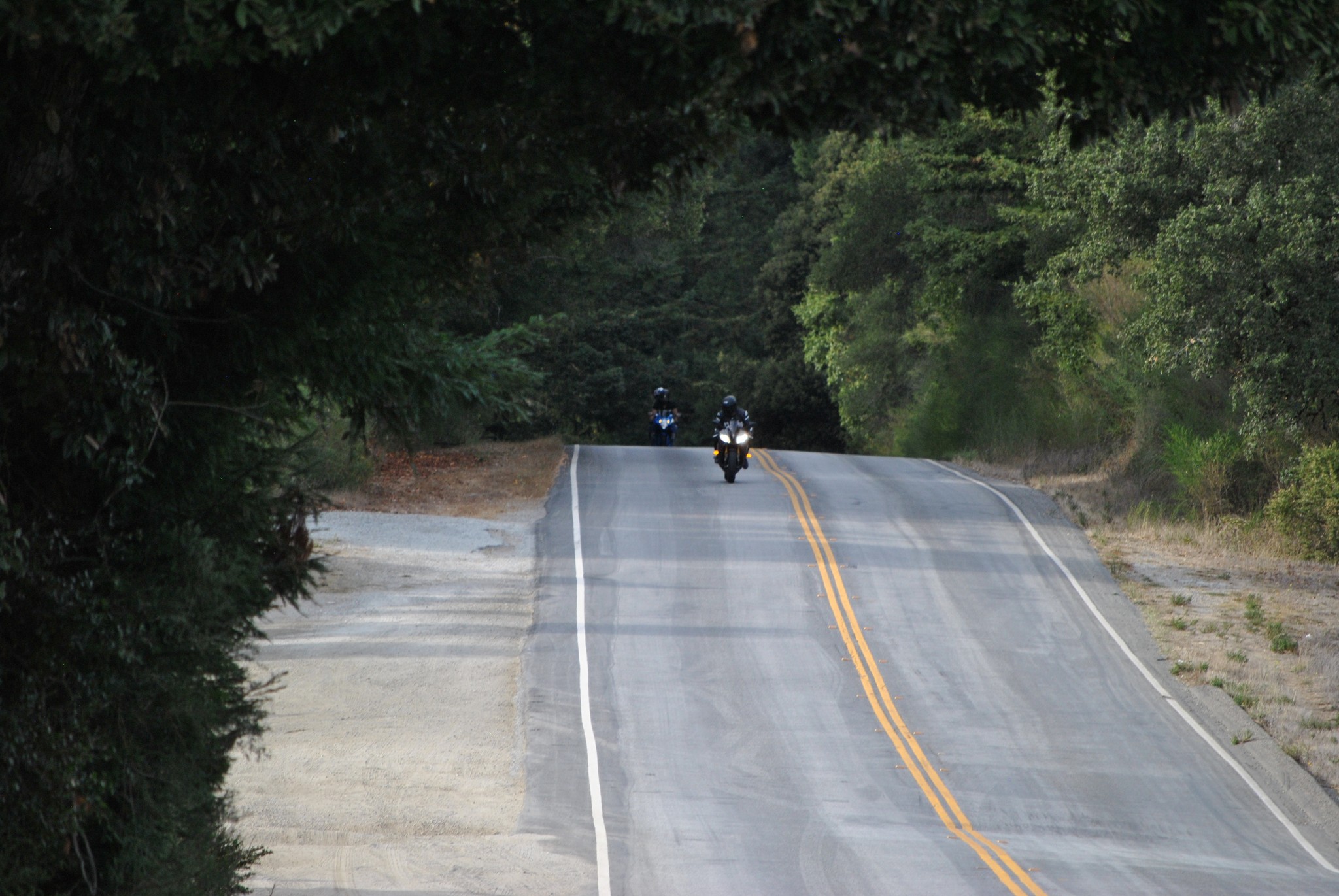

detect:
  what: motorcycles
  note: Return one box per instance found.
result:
[648,411,682,446]
[711,419,759,483]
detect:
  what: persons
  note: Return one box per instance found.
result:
[713,395,750,470]
[649,387,678,441]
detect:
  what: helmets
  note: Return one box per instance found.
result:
[653,387,664,397]
[664,389,670,399]
[722,395,737,410]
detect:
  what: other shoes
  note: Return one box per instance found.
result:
[715,455,724,463]
[743,457,748,469]
